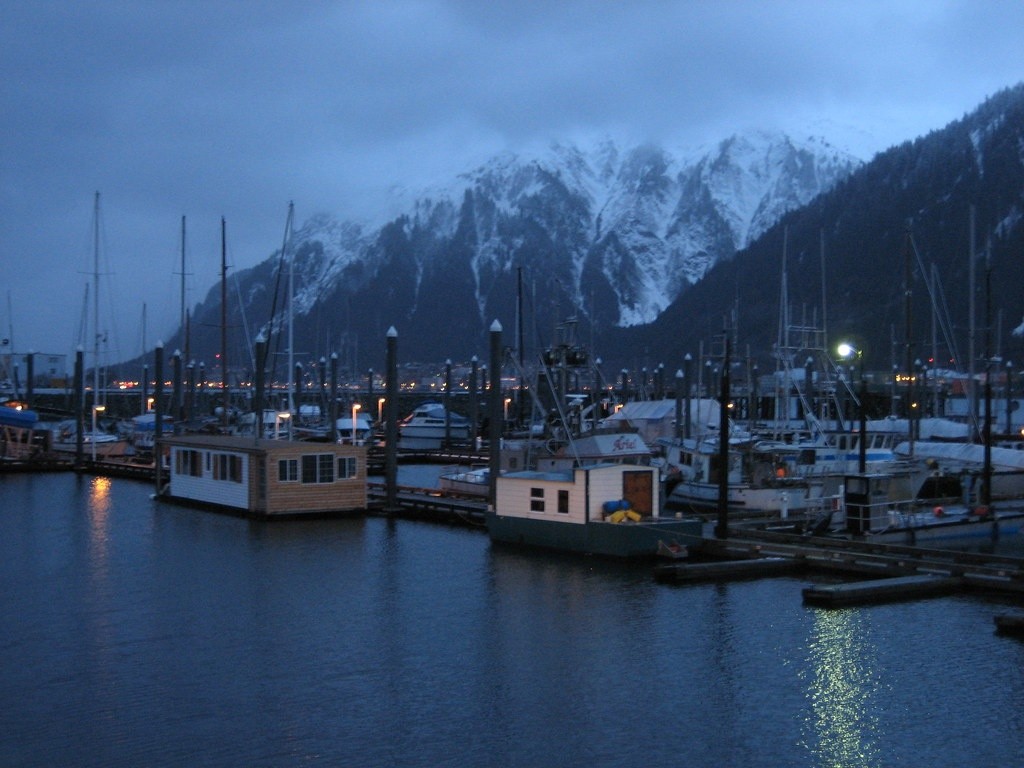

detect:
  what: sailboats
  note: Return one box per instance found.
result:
[0,190,1024,521]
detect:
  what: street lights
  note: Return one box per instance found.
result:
[836,343,866,474]
